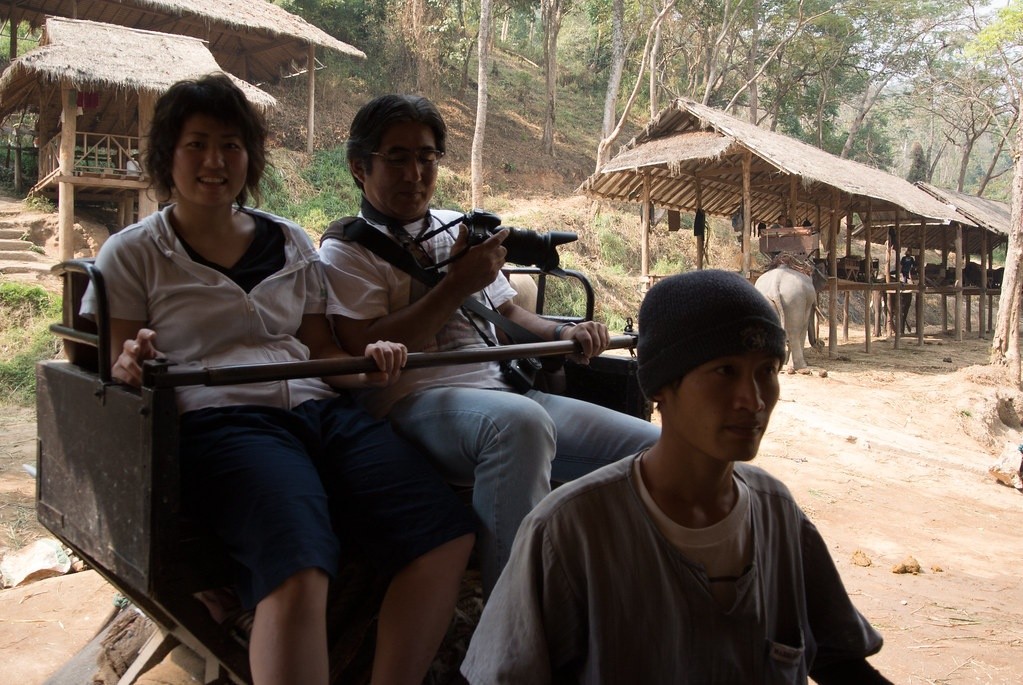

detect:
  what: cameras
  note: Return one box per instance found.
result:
[462,208,578,279]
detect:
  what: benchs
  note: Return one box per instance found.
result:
[35,256,651,685]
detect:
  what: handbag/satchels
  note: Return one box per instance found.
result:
[534,361,567,394]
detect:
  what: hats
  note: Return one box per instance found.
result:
[635,268,788,402]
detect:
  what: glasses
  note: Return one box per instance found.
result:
[369,149,445,168]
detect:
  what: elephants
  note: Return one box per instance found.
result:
[754,266,826,370]
[871,274,912,338]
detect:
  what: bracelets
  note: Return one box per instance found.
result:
[554,322,576,341]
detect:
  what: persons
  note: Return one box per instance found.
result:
[888,249,917,284]
[317,95,662,612]
[770,215,786,229]
[459,270,896,685]
[77,75,480,685]
